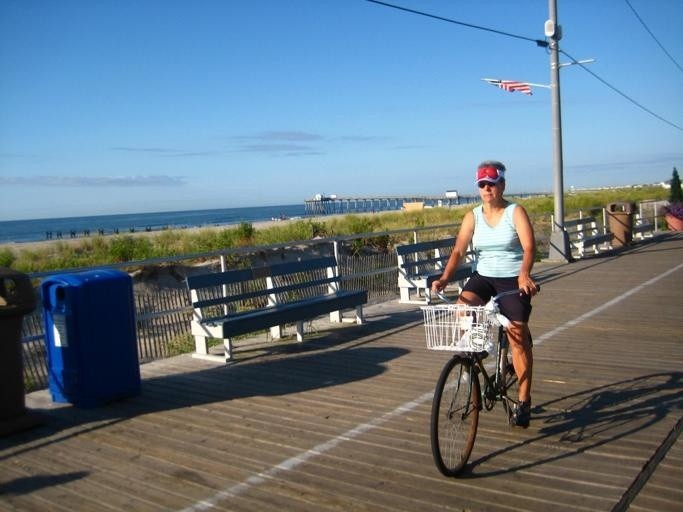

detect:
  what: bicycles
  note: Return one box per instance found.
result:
[417,284,540,477]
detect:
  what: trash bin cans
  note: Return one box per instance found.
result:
[607,201,636,246]
[0,265,139,437]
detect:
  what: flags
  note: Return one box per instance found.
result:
[488,79,533,96]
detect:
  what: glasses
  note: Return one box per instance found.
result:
[477,182,495,188]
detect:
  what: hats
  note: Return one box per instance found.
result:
[475,168,505,186]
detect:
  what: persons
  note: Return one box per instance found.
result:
[432,161,538,426]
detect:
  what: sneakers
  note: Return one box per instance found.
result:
[510,397,531,430]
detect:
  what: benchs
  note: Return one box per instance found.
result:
[391,236,478,307]
[563,217,616,261]
[179,251,370,363]
